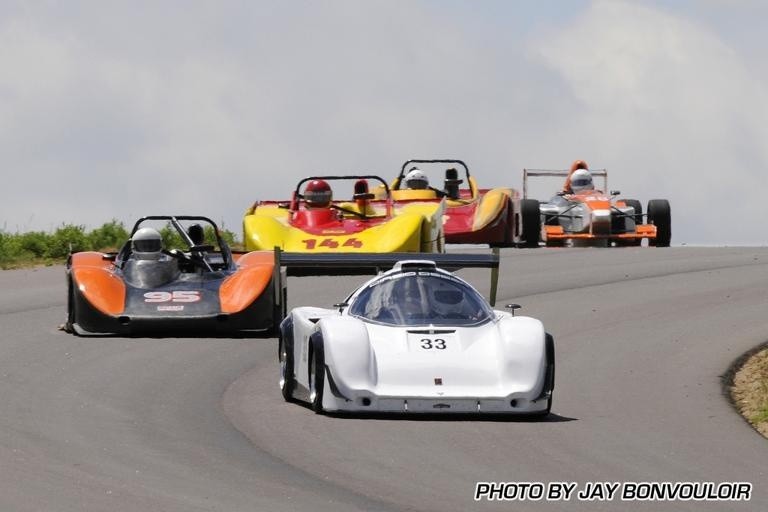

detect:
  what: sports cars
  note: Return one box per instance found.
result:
[243,175,445,269]
[390,158,521,248]
[273,247,556,420]
[61,215,287,335]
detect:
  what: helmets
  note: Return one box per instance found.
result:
[429,279,465,315]
[131,227,164,260]
[570,169,594,193]
[304,180,333,211]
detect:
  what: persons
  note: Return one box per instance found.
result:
[564,168,604,199]
[423,278,478,325]
[124,228,178,287]
[293,179,344,223]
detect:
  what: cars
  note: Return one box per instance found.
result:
[520,161,671,245]
